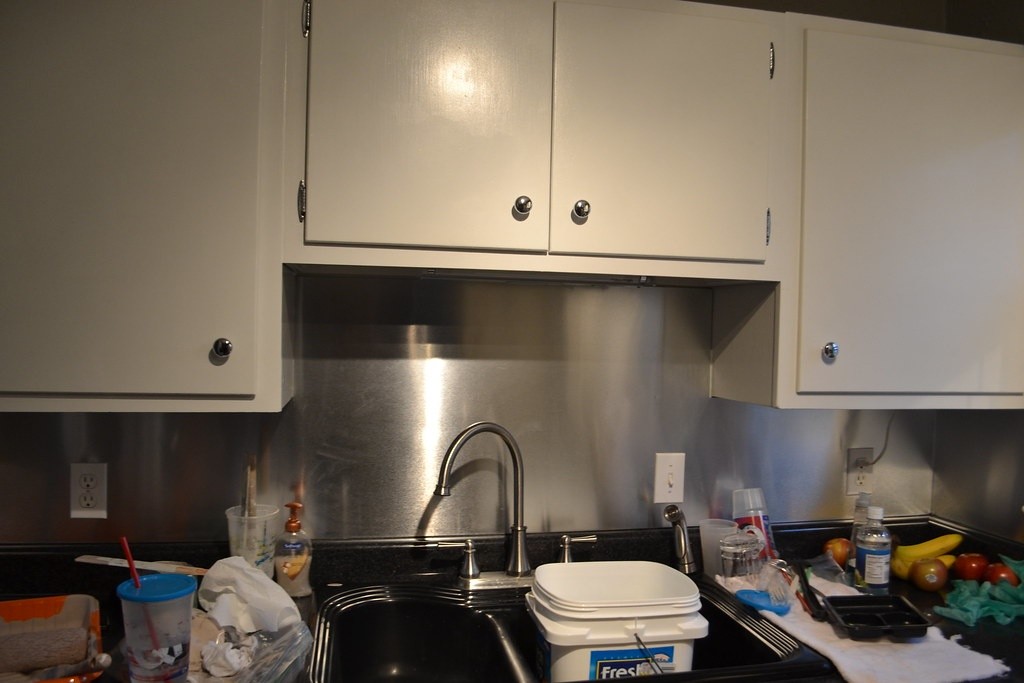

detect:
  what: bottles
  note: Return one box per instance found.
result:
[847,499,892,595]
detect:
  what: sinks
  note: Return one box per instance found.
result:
[526,579,804,683]
[310,583,527,683]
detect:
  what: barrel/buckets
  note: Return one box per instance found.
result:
[525,561,709,683]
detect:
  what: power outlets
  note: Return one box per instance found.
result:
[69,462,107,518]
[847,448,874,495]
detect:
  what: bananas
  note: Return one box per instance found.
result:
[889,533,963,580]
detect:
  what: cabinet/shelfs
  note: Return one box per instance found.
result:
[1,0,1023,410]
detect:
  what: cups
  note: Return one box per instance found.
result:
[699,487,778,578]
[224,503,280,579]
[117,574,197,683]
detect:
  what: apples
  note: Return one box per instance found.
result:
[912,557,948,591]
[953,553,1020,587]
[823,538,851,567]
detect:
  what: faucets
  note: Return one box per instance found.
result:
[434,421,533,576]
[663,504,699,573]
